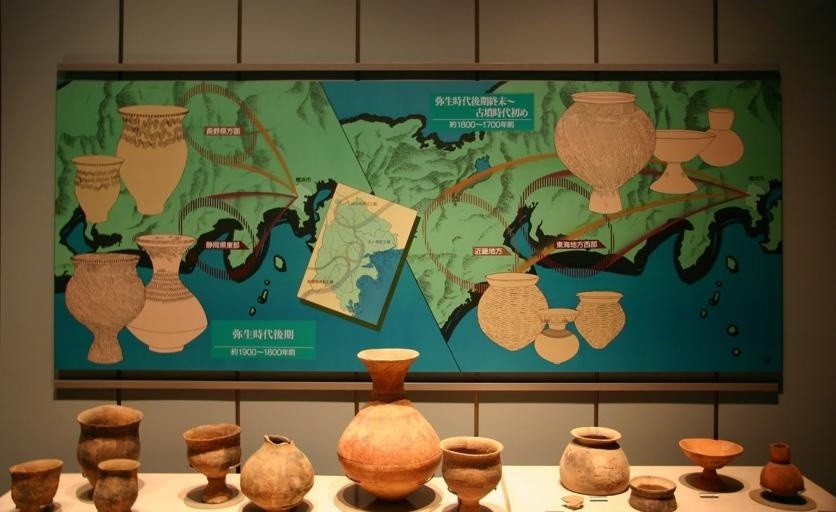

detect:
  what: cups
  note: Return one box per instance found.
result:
[76,404,144,491]
[8,458,63,512]
[93,458,139,512]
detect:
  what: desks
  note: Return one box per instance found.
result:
[0,465,836,512]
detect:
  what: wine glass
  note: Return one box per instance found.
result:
[677,437,744,493]
[440,434,505,512]
[650,129,716,196]
[181,423,242,504]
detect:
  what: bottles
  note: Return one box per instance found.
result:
[124,235,209,355]
[699,107,745,169]
[240,435,315,512]
[759,442,806,501]
[534,307,580,366]
[558,425,630,497]
